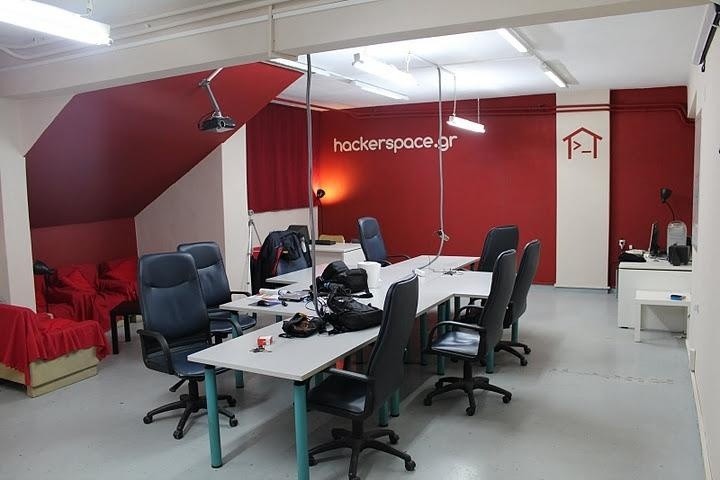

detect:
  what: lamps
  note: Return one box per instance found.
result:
[660,188,677,221]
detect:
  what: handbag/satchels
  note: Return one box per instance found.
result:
[618,253,646,262]
[282,313,324,338]
[310,261,368,294]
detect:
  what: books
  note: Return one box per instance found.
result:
[248,288,292,300]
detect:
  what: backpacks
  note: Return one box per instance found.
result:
[324,286,383,335]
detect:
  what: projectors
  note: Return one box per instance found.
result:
[197,114,238,136]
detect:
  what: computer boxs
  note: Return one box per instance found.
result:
[666,220,687,259]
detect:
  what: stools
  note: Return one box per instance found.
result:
[633,289,693,344]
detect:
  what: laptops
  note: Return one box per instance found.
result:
[309,237,337,246]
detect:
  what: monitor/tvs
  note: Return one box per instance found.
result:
[647,221,661,258]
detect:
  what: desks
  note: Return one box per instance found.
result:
[616,250,692,342]
